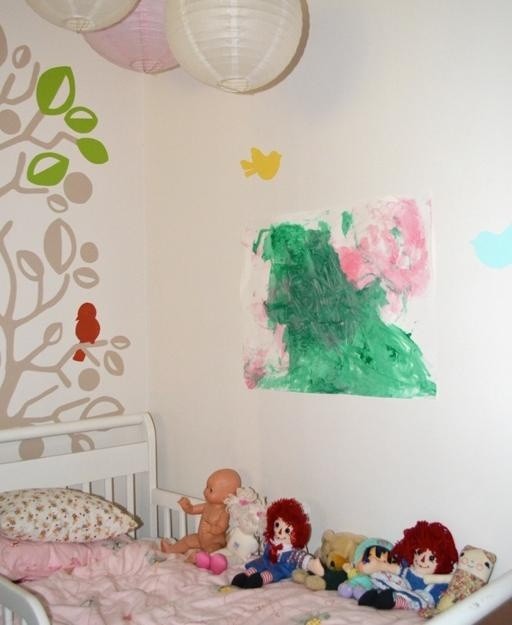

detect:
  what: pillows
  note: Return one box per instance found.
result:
[0,487,141,581]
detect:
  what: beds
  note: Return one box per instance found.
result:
[0,412,512,625]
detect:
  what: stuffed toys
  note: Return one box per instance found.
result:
[358,520,458,611]
[291,529,367,590]
[195,486,497,619]
[195,485,268,574]
[233,497,313,589]
[417,545,497,619]
[338,538,397,599]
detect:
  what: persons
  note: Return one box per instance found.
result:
[159,469,242,563]
